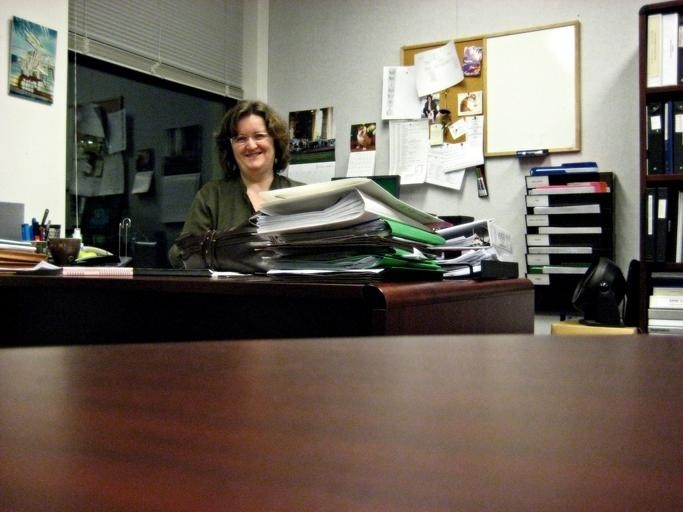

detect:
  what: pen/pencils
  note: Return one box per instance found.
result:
[517,150,548,155]
[22,209,51,241]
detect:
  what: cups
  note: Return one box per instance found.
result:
[46,238,80,267]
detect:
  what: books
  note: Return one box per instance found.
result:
[0,238,61,276]
[642,13,682,336]
[251,175,513,288]
[57,266,134,277]
[522,163,611,287]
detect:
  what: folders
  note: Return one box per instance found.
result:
[649,101,683,176]
[646,187,668,263]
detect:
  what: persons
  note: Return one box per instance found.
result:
[422,94,438,120]
[167,100,307,271]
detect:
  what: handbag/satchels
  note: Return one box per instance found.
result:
[175,211,275,274]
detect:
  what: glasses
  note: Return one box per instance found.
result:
[230,132,270,144]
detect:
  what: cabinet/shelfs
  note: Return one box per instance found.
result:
[639,0,683,335]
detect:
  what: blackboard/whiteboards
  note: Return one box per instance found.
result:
[483,20,582,157]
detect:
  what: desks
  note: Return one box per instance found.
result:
[0,271,683,512]
